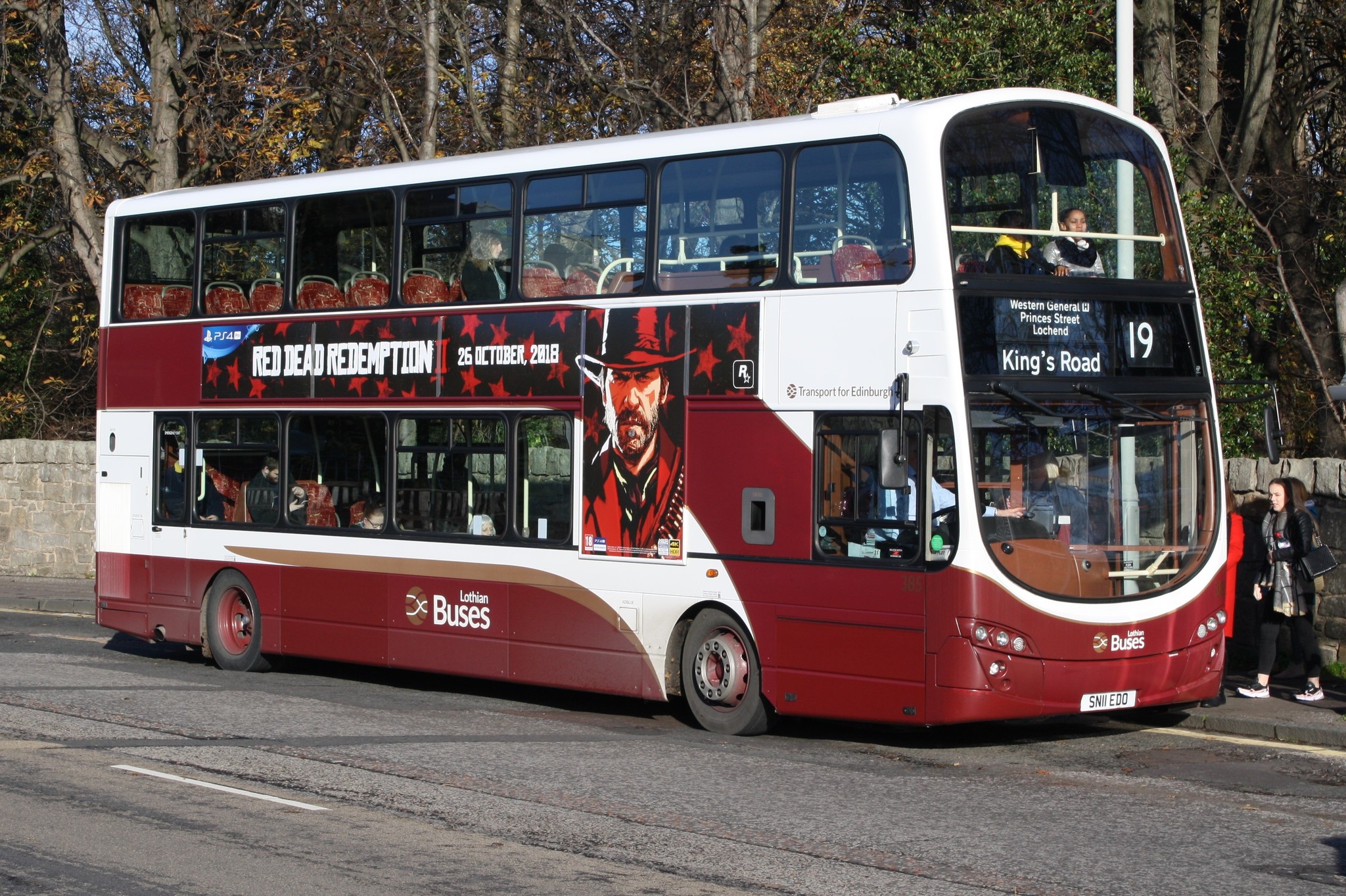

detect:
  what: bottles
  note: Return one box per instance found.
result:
[1276,533,1291,549]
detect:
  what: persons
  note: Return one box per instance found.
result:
[712,235,751,271]
[457,227,575,301]
[1225,475,1325,701]
[162,435,497,536]
[863,427,1093,550]
[986,208,1106,276]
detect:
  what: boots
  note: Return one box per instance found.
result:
[1202,648,1227,708]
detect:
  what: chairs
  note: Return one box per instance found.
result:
[122,234,1044,321]
[165,448,505,534]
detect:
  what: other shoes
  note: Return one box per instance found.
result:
[1274,663,1306,679]
[1248,661,1281,676]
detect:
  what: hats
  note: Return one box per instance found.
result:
[544,243,575,257]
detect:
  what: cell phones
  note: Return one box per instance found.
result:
[301,500,308,505]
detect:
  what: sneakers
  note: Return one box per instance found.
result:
[1236,676,1270,698]
[1293,681,1325,700]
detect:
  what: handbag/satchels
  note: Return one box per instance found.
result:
[1289,510,1338,580]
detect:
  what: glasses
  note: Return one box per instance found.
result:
[367,518,384,527]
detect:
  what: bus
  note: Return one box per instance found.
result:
[90,85,1286,738]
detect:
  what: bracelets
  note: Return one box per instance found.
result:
[994,509,997,515]
